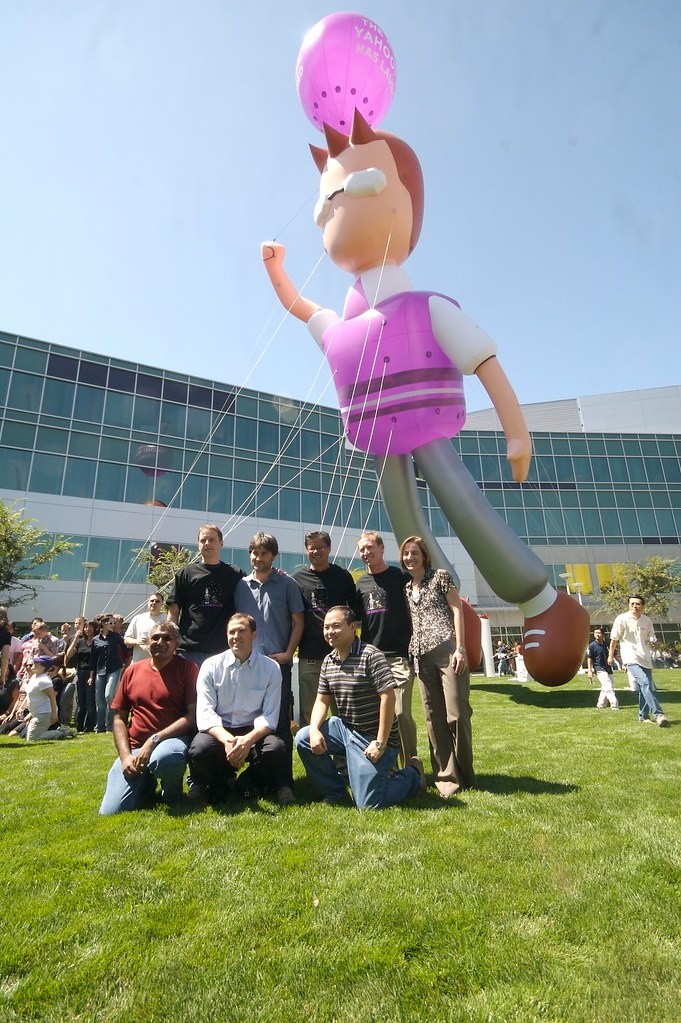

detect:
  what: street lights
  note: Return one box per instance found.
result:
[558,572,572,596]
[573,582,585,675]
[81,561,100,618]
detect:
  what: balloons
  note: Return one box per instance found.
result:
[296,13,398,135]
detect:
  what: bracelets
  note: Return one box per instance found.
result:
[456,647,466,653]
[372,740,386,751]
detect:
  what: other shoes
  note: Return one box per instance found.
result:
[321,790,352,805]
[639,719,655,724]
[187,784,206,802]
[409,755,427,793]
[186,776,193,786]
[657,714,668,727]
[276,786,296,802]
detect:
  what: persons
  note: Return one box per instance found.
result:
[649,647,681,669]
[606,596,668,726]
[492,641,523,677]
[99,621,201,811]
[233,530,303,753]
[261,106,590,684]
[290,531,360,728]
[400,535,477,800]
[186,613,289,803]
[294,606,429,810]
[352,530,418,769]
[586,626,620,711]
[0,606,134,741]
[123,593,180,665]
[165,525,287,670]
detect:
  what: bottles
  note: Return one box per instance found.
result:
[590,678,594,686]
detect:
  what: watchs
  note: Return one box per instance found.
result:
[151,733,160,743]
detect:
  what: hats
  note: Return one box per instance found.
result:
[32,655,53,668]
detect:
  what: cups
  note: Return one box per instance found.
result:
[650,635,658,646]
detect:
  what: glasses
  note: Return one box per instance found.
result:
[151,634,172,642]
[105,621,115,626]
[308,546,325,553]
[150,599,160,603]
